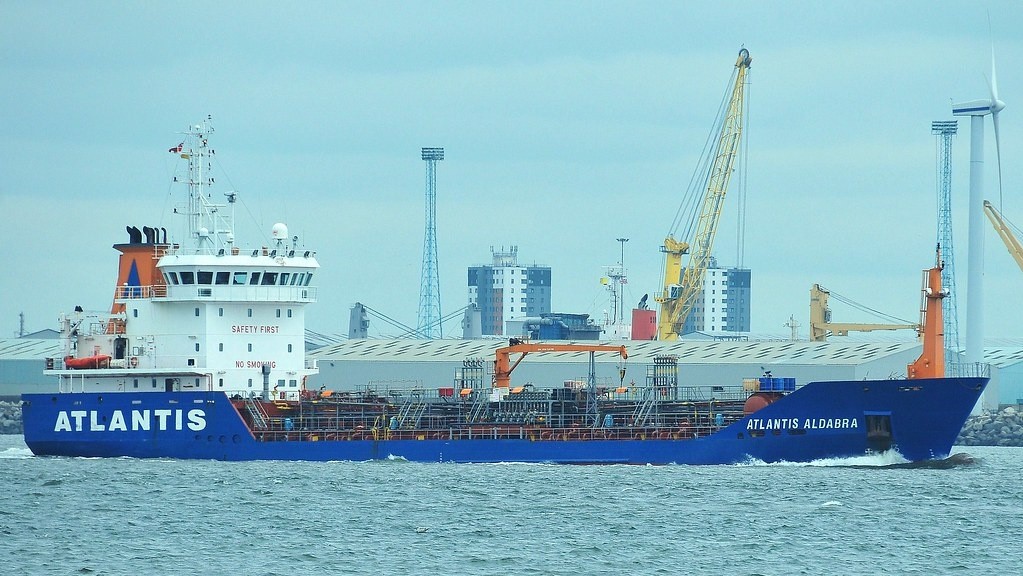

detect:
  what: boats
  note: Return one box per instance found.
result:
[19,118,997,471]
[64,354,111,370]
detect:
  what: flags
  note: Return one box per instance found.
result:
[169,143,184,153]
[621,278,627,284]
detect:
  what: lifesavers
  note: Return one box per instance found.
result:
[130,356,138,366]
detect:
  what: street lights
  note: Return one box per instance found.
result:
[616,237,631,321]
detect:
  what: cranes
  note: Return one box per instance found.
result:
[980,198,1023,273]
[806,283,923,343]
[493,334,630,404]
[653,46,753,342]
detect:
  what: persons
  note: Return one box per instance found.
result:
[116,340,124,359]
